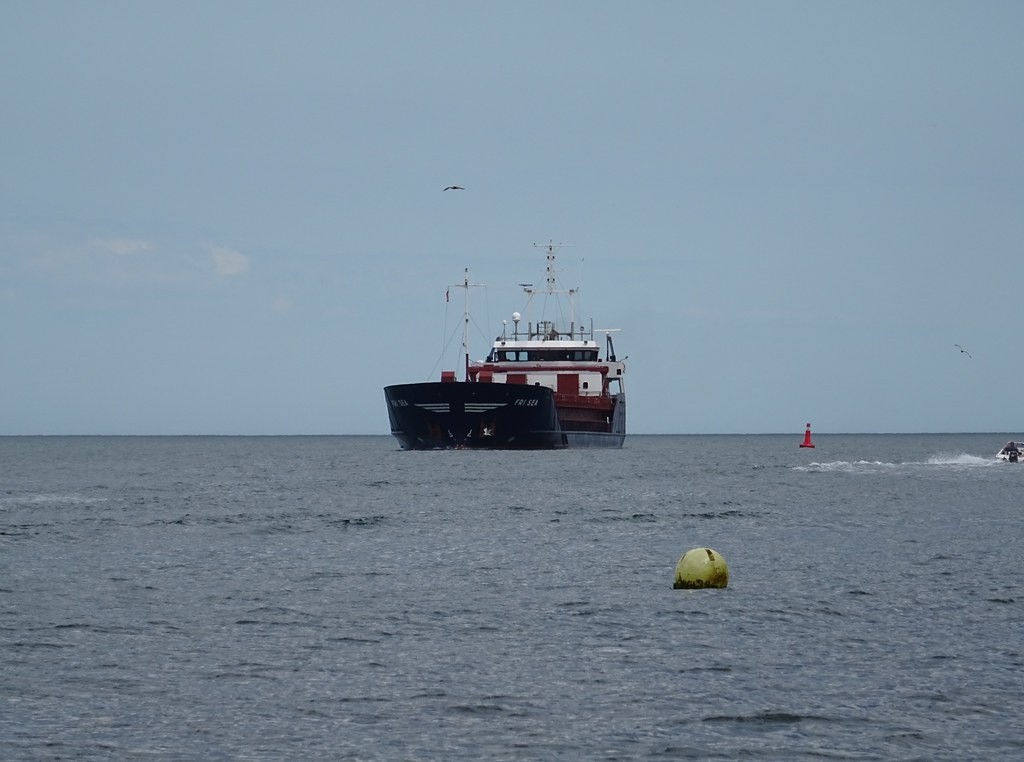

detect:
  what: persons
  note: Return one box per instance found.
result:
[1004,441,1021,455]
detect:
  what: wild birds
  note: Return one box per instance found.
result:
[953,343,972,359]
[442,185,465,191]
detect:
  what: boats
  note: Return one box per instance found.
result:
[996,441,1023,464]
[382,236,630,452]
